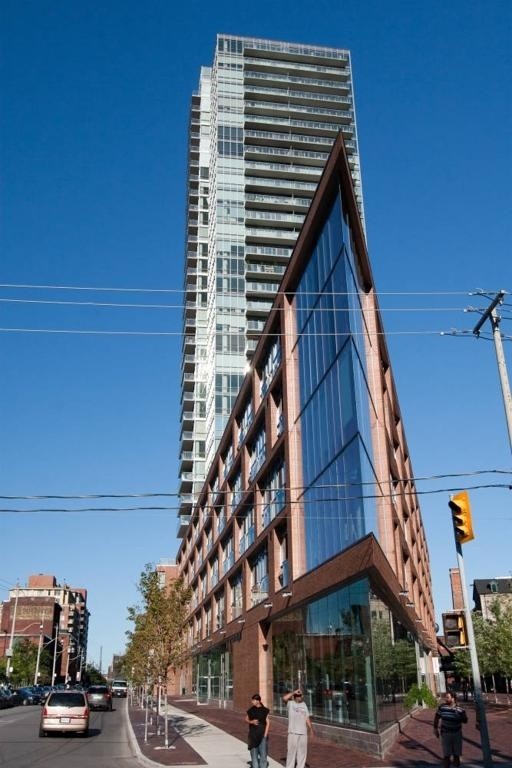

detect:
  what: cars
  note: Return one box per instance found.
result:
[38,689,89,739]
[85,684,113,710]
[0,682,86,708]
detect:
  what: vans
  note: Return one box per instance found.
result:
[110,679,127,698]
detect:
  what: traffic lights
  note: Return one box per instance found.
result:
[448,489,475,545]
[442,612,468,648]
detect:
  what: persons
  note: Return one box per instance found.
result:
[244,693,270,768]
[433,691,468,768]
[282,688,314,768]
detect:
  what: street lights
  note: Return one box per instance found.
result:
[7,581,85,688]
